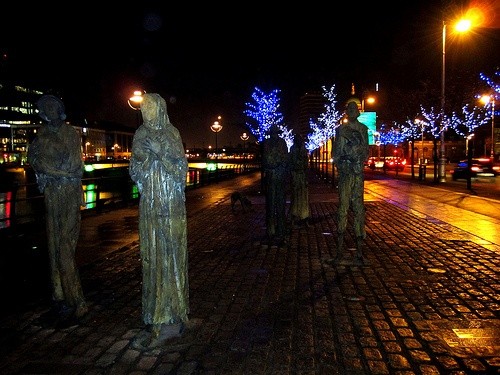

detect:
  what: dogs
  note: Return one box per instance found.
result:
[231,192,253,211]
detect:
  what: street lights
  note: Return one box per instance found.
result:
[127,88,148,128]
[112,144,120,158]
[211,119,223,173]
[240,132,249,166]
[438,8,482,184]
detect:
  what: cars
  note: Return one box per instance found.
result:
[369,156,405,171]
[456,157,497,178]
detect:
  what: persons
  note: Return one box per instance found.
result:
[25,95,88,316]
[261,124,289,239]
[330,100,369,250]
[130,93,188,327]
[288,134,310,220]
